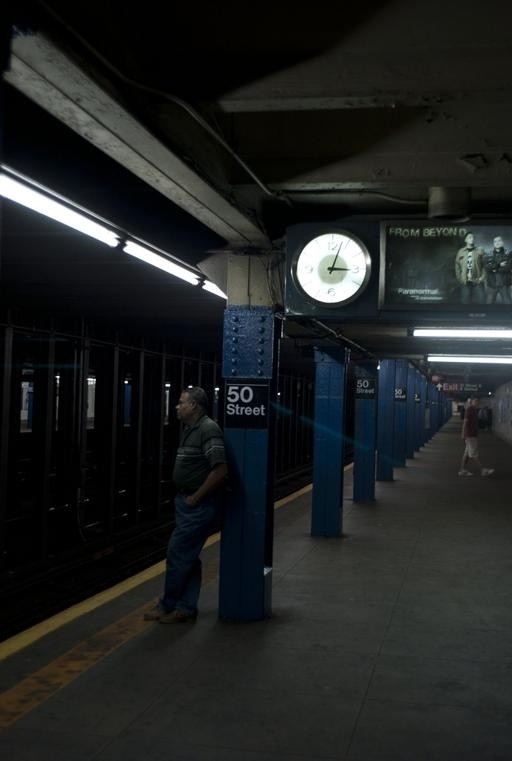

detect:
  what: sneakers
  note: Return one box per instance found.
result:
[456,468,496,477]
[143,603,198,625]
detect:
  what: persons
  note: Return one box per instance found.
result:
[457,395,495,478]
[144,385,229,626]
[453,232,487,304]
[480,234,512,305]
[460,406,492,433]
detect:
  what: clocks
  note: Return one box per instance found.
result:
[290,230,373,311]
